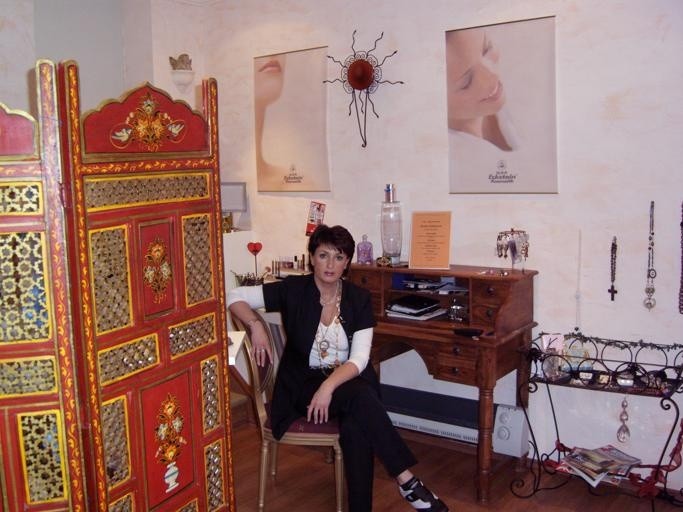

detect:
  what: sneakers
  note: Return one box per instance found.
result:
[400,476,449,512]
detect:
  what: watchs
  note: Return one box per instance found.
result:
[247,318,258,329]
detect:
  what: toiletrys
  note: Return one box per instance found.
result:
[270,254,306,276]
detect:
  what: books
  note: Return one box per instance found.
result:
[384,294,448,321]
[555,444,642,488]
[541,334,593,378]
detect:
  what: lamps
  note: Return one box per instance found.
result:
[166,52,195,94]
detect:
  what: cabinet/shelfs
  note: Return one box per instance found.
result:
[321,261,538,502]
[508,332,683,510]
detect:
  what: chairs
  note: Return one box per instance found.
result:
[228,308,347,512]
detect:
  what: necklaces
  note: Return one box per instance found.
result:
[644,200,657,309]
[679,201,683,315]
[314,280,343,377]
[320,280,339,306]
[608,236,618,301]
[320,307,336,358]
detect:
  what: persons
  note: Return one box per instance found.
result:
[225,224,449,512]
[253,53,318,191]
[445,26,527,193]
